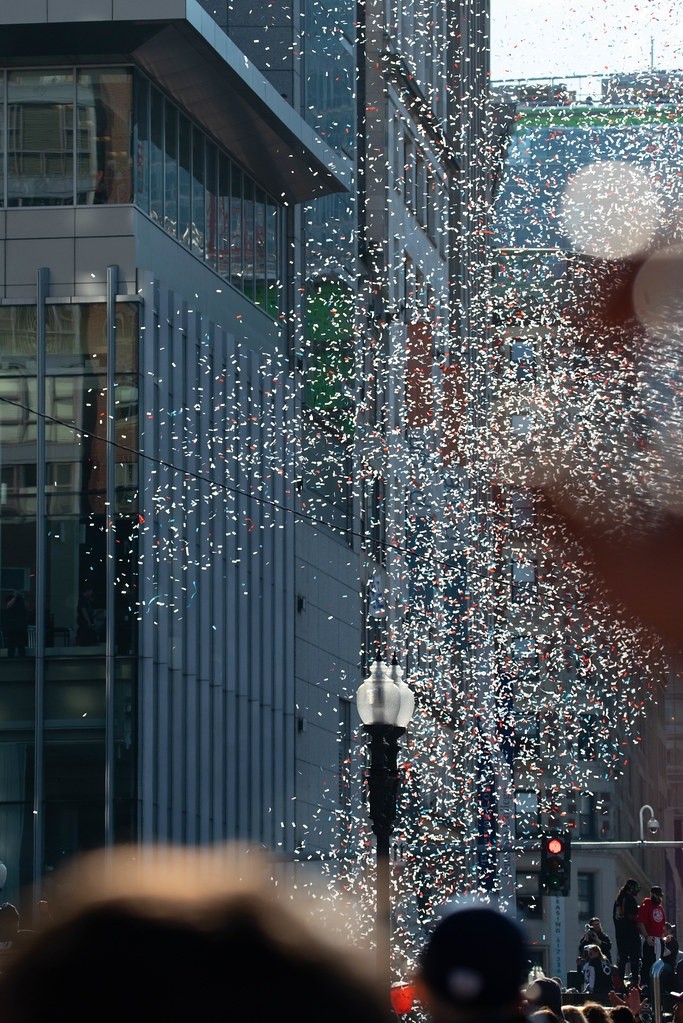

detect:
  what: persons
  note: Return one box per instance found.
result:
[516,905,683,1023]
[413,908,533,1023]
[612,879,643,993]
[577,916,612,967]
[635,885,676,992]
[585,944,613,1000]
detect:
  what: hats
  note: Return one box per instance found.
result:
[525,978,562,1005]
[650,886,663,896]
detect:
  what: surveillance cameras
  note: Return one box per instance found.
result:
[648,820,659,832]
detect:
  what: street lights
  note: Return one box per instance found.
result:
[637,801,659,903]
[355,645,418,1023]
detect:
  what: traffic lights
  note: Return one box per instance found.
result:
[538,828,571,898]
